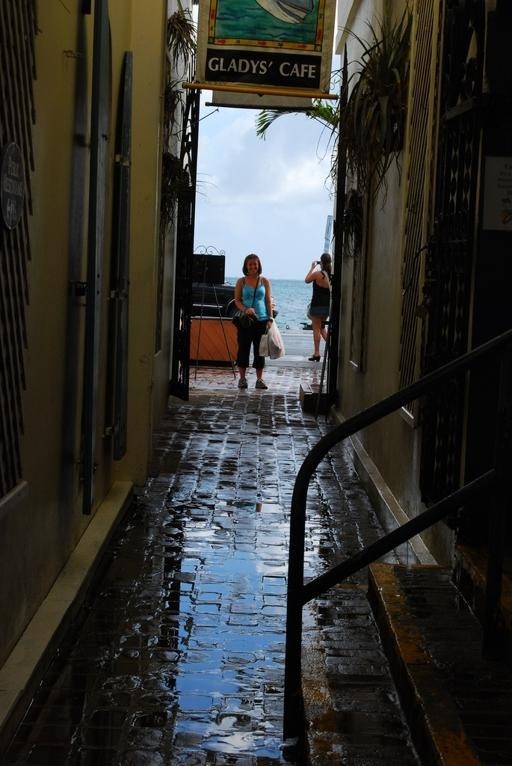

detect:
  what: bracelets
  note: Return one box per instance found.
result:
[244,307,249,313]
[268,316,273,322]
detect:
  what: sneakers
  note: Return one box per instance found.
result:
[237,377,248,388]
[255,379,267,389]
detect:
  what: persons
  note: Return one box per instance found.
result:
[305,252,333,361]
[234,253,273,388]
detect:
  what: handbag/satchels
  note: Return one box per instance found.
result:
[259,328,271,359]
[232,309,259,331]
[306,304,312,320]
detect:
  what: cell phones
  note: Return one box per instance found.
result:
[317,261,320,264]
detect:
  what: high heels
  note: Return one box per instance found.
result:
[308,355,320,361]
